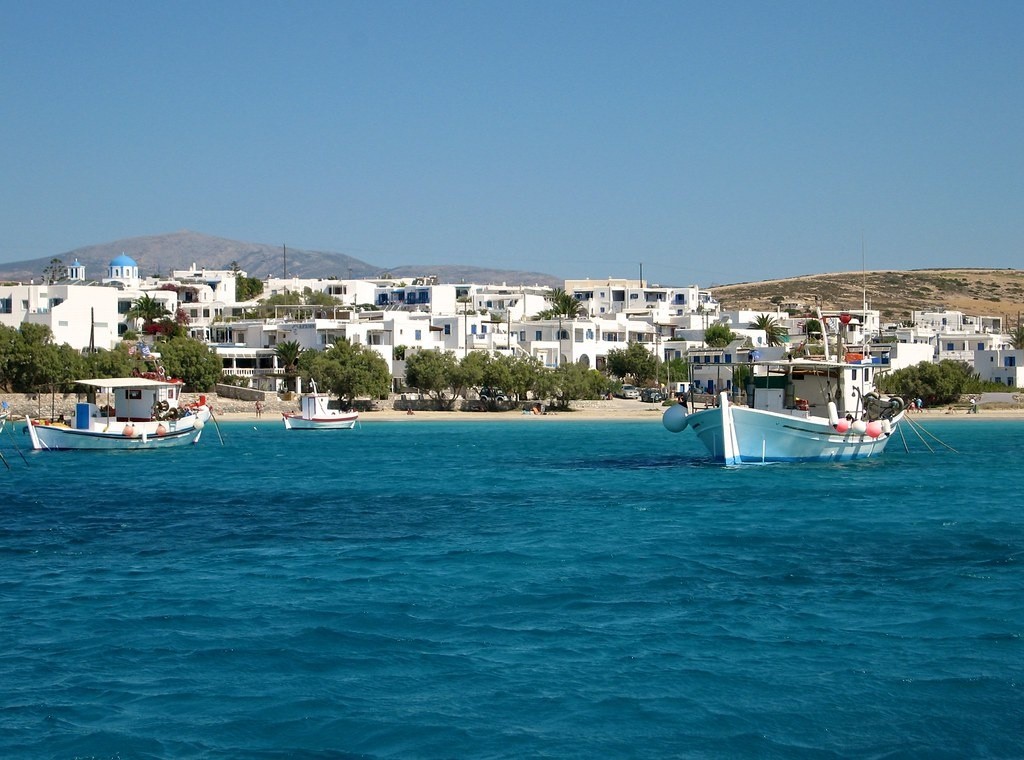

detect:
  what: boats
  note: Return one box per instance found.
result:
[663,230,923,466]
[281,378,359,430]
[23,340,212,450]
[0,401,12,433]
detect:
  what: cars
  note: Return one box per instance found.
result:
[642,389,663,403]
[616,384,639,398]
[479,387,506,401]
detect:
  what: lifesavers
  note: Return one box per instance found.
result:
[153,402,162,414]
[157,366,166,376]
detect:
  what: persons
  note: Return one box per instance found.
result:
[407,408,415,415]
[909,397,922,413]
[967,395,976,414]
[58,415,66,423]
[256,399,262,419]
[531,406,549,415]
